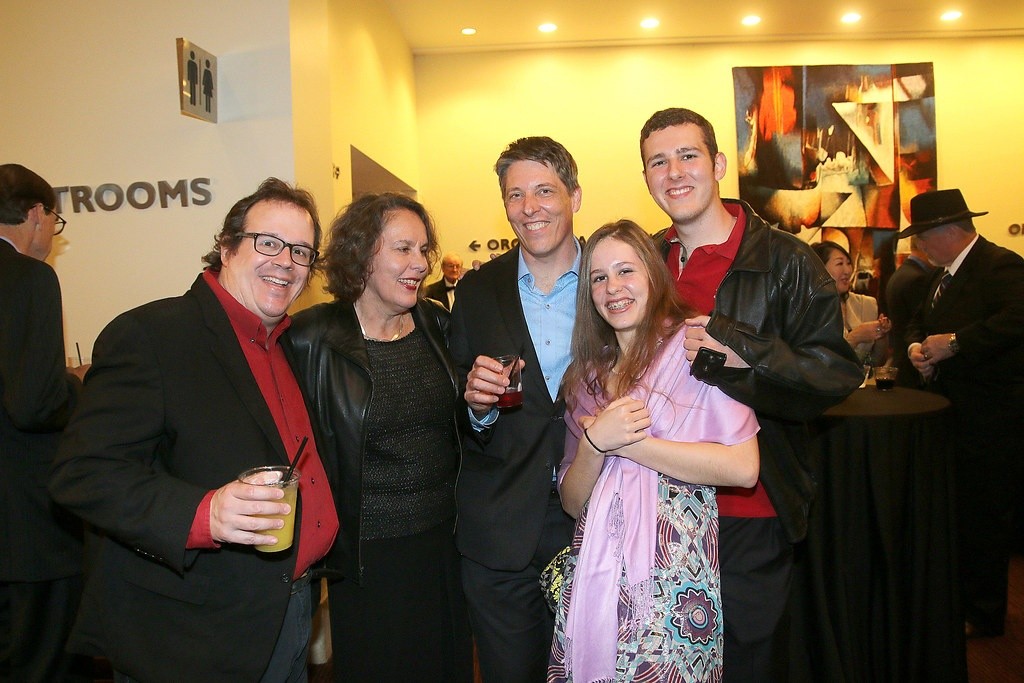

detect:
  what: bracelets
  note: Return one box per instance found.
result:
[584,429,607,453]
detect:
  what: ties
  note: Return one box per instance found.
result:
[926,269,954,317]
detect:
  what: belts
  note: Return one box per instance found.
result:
[290,569,317,595]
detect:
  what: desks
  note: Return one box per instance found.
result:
[791,387,970,683]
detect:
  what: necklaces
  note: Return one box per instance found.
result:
[364,314,405,342]
[612,346,629,375]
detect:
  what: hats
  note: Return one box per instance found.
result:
[895,189,989,240]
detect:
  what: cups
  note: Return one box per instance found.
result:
[875,366,898,392]
[493,355,524,409]
[67,355,91,368]
[237,465,301,552]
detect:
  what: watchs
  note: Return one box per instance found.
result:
[948,333,961,354]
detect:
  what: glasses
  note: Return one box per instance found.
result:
[234,231,320,268]
[30,204,67,236]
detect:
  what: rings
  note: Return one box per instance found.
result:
[876,328,880,332]
[925,354,928,360]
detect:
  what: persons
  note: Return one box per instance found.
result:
[882,235,936,388]
[447,131,605,683]
[891,187,1024,635]
[636,108,867,682]
[545,219,763,683]
[50,175,342,682]
[812,241,892,368]
[0,162,88,683]
[281,186,476,683]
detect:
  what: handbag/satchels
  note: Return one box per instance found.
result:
[538,543,575,615]
